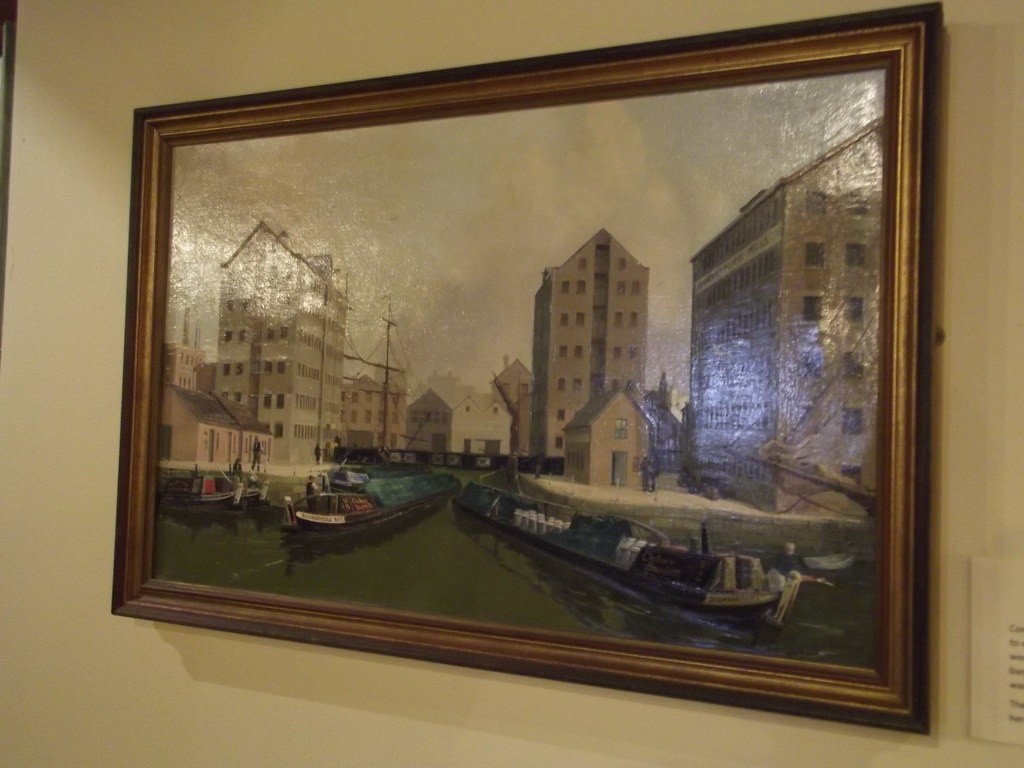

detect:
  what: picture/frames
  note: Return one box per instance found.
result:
[111,2,945,736]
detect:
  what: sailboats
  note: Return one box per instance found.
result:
[277,291,465,537]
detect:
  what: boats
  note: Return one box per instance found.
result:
[156,468,265,512]
[448,476,800,610]
